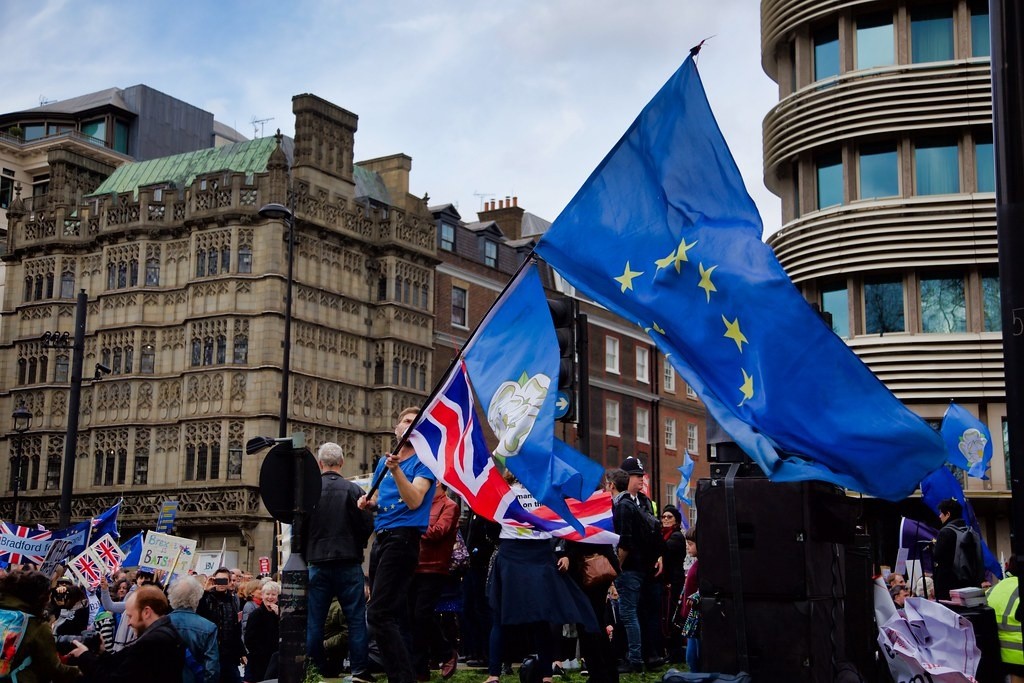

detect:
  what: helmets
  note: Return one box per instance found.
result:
[57,577,73,585]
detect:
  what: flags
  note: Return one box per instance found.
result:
[900,518,939,574]
[676,450,692,505]
[939,404,993,480]
[463,263,606,535]
[408,362,620,545]
[534,58,945,497]
[677,501,689,531]
[119,535,143,567]
[921,468,1003,579]
[45,519,90,557]
[88,502,120,545]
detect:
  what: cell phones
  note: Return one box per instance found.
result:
[214,578,228,585]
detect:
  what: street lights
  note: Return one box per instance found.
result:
[256,194,295,437]
[11,400,33,524]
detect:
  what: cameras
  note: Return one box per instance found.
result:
[56,631,101,659]
[55,590,70,601]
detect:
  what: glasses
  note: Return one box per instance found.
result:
[662,515,674,519]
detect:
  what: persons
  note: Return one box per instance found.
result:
[889,503,1024,680]
[0,442,386,683]
[358,407,437,683]
[416,460,699,683]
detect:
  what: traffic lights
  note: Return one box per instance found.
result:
[546,298,576,423]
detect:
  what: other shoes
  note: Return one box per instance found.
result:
[467,660,487,666]
[543,677,551,683]
[352,669,377,683]
[484,676,499,683]
[562,659,570,670]
[552,664,566,677]
[569,658,578,670]
[580,667,589,674]
[618,662,643,674]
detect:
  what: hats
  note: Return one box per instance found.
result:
[661,508,682,524]
[621,455,645,475]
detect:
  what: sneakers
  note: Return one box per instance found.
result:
[442,651,457,679]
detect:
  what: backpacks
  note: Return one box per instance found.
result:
[940,523,985,587]
[0,609,35,683]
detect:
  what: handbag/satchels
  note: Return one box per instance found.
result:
[581,553,617,591]
